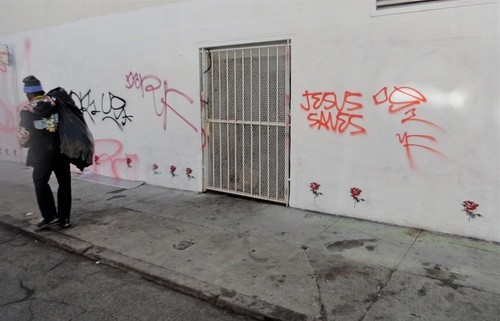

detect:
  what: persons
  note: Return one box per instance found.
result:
[15,74,74,231]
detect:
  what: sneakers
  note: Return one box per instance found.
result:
[37,213,58,229]
[55,218,71,227]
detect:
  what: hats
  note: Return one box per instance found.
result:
[23,75,42,92]
